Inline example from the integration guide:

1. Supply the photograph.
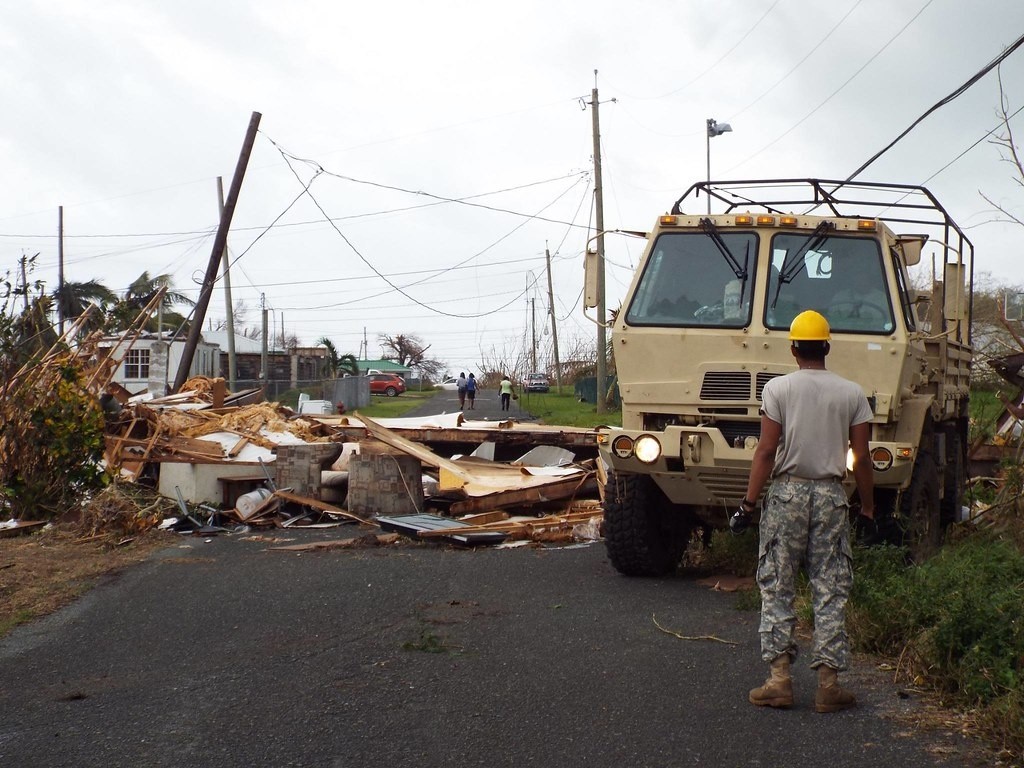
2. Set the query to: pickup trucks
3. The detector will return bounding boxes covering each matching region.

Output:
[523,373,550,393]
[342,370,383,378]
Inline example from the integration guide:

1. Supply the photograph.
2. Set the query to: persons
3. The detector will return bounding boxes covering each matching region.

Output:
[498,377,518,411]
[730,310,878,712]
[457,372,479,410]
[826,251,890,322]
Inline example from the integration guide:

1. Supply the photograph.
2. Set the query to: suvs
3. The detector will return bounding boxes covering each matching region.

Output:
[365,374,407,396]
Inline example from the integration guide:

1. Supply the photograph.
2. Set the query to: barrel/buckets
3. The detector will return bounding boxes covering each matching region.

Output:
[236,487,272,518]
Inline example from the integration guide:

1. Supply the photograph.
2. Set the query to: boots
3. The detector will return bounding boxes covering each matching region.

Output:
[749,655,793,707]
[815,665,856,712]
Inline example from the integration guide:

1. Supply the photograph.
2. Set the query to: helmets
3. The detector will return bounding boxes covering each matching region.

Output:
[787,310,831,340]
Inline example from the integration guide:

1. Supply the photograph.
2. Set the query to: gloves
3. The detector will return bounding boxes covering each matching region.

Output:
[853,514,875,542]
[729,505,754,536]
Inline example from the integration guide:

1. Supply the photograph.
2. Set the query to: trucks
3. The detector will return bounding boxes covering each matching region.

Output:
[584,176,975,578]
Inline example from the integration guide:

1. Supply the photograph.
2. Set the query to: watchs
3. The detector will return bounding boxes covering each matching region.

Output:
[742,496,756,507]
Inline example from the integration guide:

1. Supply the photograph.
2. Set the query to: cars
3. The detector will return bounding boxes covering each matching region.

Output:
[432,378,459,390]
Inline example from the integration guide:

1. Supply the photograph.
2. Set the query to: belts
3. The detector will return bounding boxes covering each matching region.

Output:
[778,473,834,483]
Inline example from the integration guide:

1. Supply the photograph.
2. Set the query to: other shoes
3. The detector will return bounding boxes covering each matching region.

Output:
[468,407,470,410]
[502,406,504,410]
[471,408,475,410]
[460,406,463,410]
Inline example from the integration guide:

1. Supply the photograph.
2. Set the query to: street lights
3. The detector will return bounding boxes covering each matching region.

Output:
[705,118,734,214]
